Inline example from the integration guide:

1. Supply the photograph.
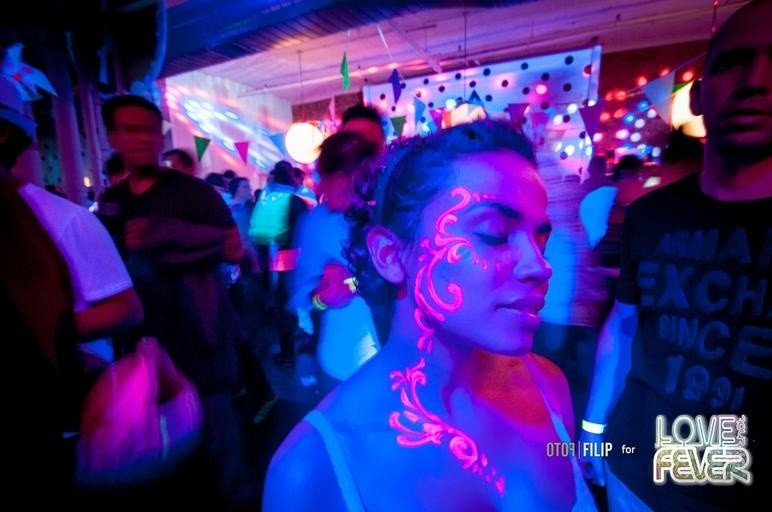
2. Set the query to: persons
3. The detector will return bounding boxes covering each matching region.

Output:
[1,0,772,511]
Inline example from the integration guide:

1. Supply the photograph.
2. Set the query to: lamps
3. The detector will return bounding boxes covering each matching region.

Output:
[284,51,323,163]
[442,12,488,128]
